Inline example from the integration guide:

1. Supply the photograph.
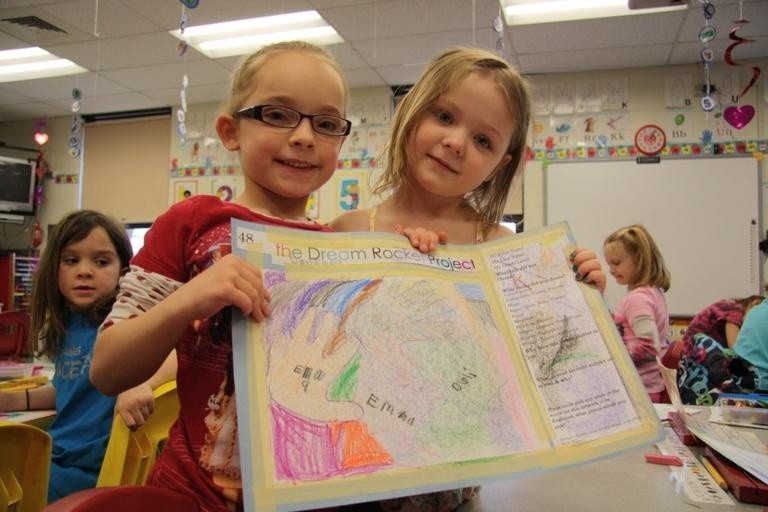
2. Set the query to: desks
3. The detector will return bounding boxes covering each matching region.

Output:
[471,403,767,512]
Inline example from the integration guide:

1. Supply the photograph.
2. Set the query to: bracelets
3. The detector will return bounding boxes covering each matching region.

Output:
[25,388,31,413]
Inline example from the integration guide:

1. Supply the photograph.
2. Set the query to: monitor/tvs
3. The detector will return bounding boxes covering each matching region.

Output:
[0,156,37,212]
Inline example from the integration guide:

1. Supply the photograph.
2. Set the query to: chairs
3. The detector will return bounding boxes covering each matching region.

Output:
[0,378,201,512]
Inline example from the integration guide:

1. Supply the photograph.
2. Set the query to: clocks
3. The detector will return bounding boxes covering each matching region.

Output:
[635,124,666,156]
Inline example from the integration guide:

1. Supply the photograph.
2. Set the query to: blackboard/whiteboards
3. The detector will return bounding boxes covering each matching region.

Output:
[545,156,760,316]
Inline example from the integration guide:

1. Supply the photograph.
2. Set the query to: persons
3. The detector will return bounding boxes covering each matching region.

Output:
[690,227,768,410]
[602,222,673,403]
[90,38,449,512]
[0,210,181,503]
[673,293,768,406]
[323,42,611,512]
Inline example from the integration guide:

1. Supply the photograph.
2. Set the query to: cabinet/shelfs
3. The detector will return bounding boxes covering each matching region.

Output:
[0,253,41,334]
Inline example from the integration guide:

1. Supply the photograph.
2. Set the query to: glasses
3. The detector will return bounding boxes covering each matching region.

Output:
[232,103,351,137]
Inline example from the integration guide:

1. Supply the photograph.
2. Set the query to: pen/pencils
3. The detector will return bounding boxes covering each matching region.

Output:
[697,454,728,494]
